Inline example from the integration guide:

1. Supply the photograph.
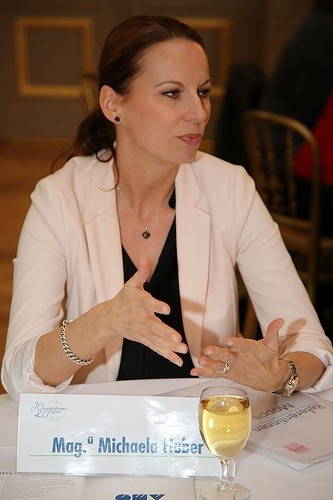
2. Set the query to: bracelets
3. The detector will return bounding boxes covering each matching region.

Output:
[59,319,95,365]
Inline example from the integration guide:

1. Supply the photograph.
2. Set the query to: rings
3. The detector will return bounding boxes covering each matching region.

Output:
[222,363,231,379]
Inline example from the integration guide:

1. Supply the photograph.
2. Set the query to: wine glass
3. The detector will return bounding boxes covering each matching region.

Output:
[198,386,251,500]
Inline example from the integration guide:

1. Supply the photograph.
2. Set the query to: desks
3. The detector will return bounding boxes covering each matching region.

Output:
[0,378,333,500]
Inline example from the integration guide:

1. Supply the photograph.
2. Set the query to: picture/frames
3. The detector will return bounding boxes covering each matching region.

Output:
[177,17,231,99]
[14,16,95,99]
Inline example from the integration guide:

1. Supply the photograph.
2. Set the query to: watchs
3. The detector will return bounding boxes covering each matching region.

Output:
[271,358,299,398]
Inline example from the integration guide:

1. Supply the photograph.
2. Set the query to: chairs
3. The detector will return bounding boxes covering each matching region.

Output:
[241,108,333,340]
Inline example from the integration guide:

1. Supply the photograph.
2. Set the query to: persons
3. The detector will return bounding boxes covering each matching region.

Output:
[1,13,332,405]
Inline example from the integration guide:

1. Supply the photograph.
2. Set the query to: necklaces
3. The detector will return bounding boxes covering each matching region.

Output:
[115,177,176,239]
